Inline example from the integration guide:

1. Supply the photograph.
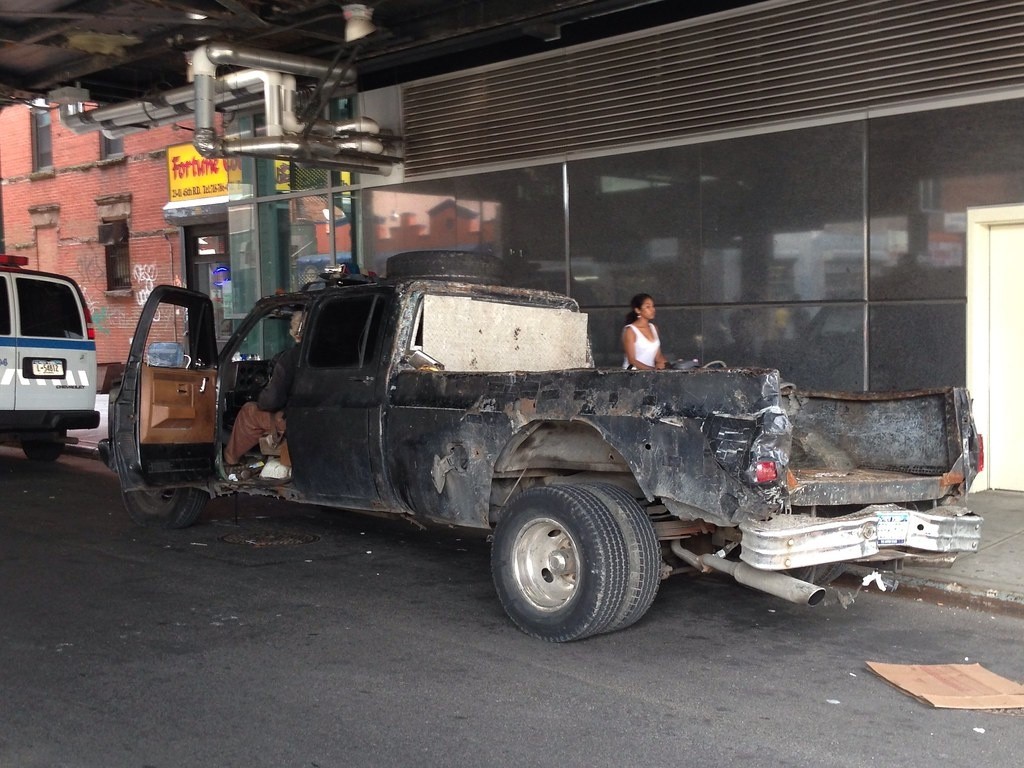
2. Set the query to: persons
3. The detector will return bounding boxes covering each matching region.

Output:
[220,310,307,468]
[621,294,669,370]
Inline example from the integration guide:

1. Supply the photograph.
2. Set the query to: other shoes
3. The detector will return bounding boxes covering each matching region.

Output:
[221,454,241,465]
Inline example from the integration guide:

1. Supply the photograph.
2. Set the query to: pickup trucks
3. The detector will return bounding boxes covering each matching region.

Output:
[97,250,987,645]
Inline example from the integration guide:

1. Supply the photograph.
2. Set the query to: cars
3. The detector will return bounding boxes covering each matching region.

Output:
[0,254,100,462]
